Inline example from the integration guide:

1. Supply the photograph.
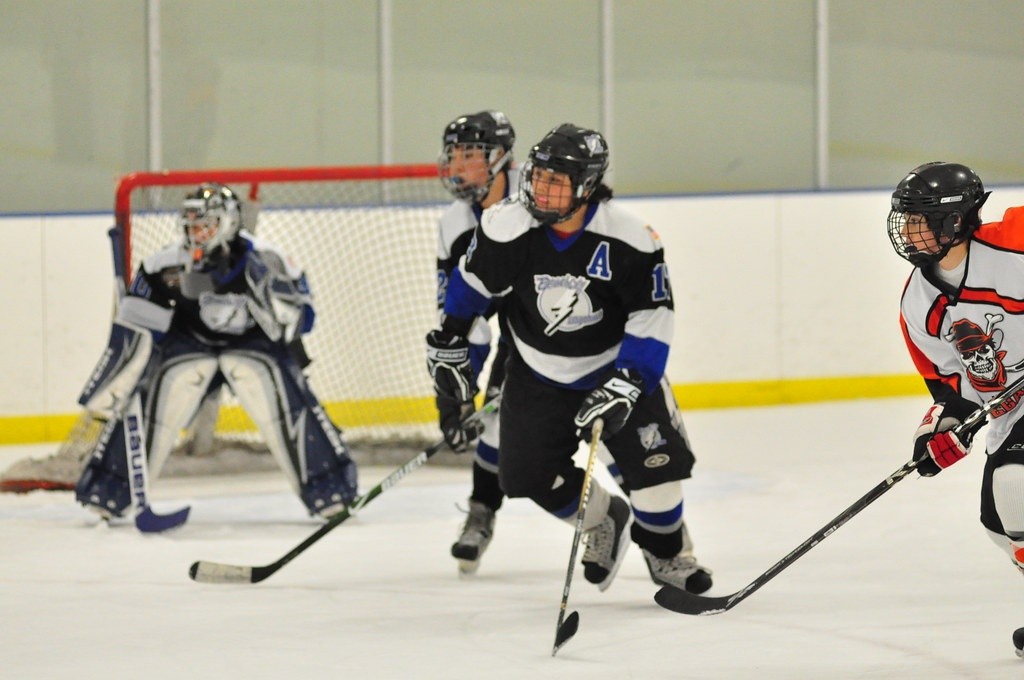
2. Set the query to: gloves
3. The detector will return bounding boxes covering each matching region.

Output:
[436,400,484,453]
[910,398,988,477]
[423,332,471,400]
[574,375,643,440]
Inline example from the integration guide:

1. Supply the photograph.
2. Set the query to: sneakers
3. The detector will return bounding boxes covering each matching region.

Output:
[452,495,495,574]
[643,550,714,595]
[582,493,635,592]
[1013,627,1024,656]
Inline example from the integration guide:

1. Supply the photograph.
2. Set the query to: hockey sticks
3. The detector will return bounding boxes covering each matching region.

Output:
[651,373,1024,619]
[550,416,606,659]
[186,394,502,589]
[107,223,192,535]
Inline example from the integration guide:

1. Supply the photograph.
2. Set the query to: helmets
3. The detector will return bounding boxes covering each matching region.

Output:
[438,110,514,202]
[177,183,240,260]
[887,162,991,269]
[518,123,609,223]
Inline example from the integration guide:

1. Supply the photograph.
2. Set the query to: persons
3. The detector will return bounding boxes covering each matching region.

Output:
[72,184,359,528]
[888,161,1024,661]
[426,123,713,594]
[437,111,631,580]
